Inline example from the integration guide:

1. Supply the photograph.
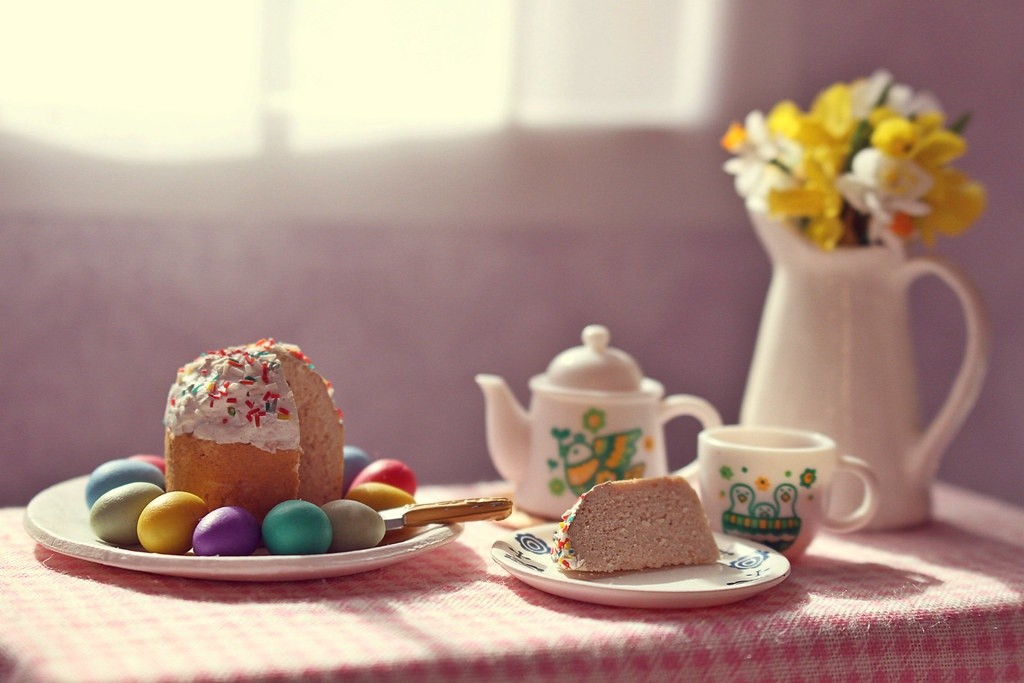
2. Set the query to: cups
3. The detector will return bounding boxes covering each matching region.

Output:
[696,425,881,562]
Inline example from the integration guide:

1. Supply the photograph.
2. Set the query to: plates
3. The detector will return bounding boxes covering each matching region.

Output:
[490,521,791,609]
[21,475,465,582]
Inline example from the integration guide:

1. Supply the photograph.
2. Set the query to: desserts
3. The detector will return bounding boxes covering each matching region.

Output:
[161,339,345,514]
[550,477,722,572]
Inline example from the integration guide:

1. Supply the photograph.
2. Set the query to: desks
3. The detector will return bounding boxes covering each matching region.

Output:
[1,482,1023,683]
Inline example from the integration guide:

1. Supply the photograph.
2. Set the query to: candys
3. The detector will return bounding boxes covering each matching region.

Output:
[84,446,418,557]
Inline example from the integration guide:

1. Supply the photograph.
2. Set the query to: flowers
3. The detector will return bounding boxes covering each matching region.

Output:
[718,71,990,249]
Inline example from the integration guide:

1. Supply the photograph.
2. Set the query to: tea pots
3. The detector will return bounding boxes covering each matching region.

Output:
[473,324,724,520]
